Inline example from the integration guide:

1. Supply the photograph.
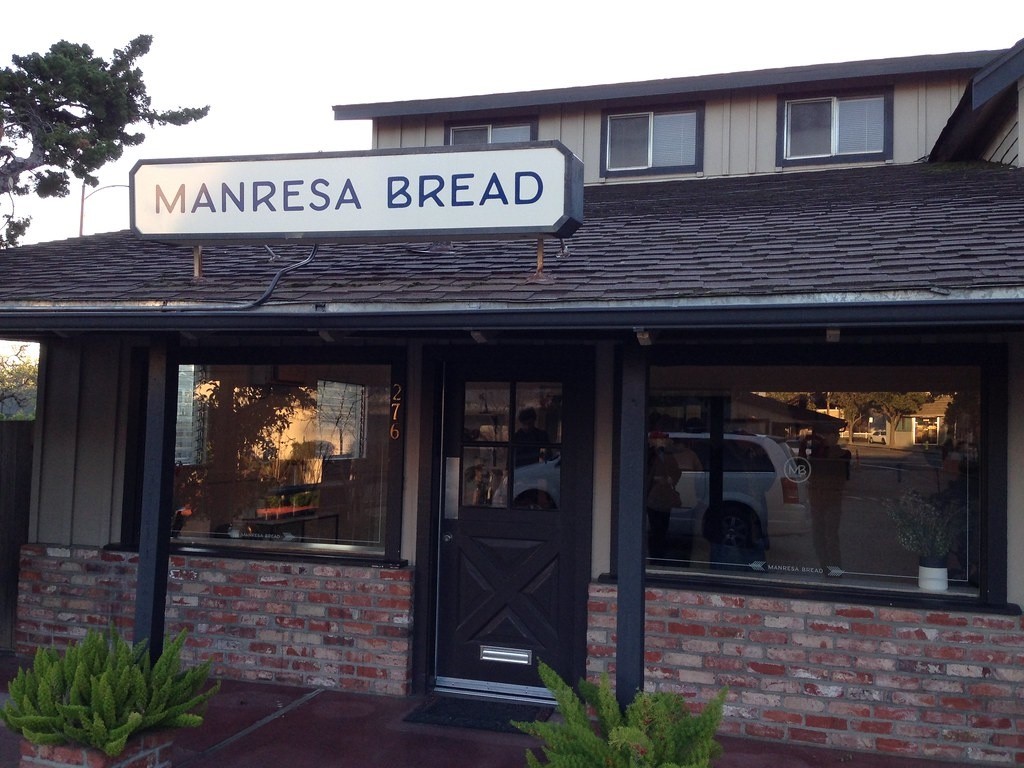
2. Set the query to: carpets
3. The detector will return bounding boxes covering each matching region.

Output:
[403,697,554,734]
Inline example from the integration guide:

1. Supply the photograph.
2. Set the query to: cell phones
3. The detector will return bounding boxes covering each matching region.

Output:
[806,435,818,440]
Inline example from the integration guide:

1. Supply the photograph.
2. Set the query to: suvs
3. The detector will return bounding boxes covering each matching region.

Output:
[489,431,811,564]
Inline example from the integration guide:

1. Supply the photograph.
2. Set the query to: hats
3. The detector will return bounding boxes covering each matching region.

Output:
[650,431,669,439]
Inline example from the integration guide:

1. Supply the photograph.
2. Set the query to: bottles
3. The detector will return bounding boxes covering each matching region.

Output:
[476,463,487,505]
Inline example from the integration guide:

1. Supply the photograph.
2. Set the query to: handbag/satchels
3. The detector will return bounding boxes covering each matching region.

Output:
[668,488,682,507]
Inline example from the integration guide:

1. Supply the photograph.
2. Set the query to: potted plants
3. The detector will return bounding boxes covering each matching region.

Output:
[878,491,972,592]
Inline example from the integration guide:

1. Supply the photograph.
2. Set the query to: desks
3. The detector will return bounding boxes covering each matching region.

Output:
[231,511,340,543]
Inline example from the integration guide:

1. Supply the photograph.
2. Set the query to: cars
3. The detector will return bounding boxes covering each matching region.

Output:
[868,430,888,445]
[787,439,812,456]
[173,461,239,519]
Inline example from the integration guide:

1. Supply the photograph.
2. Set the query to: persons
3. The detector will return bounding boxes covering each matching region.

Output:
[795,424,853,577]
[647,427,705,568]
[504,407,557,510]
